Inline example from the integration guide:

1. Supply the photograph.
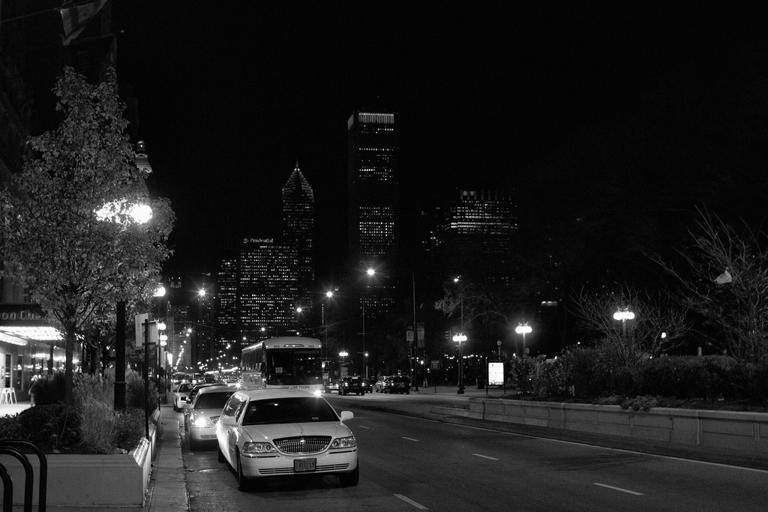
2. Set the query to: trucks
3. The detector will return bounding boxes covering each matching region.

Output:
[656,327,727,358]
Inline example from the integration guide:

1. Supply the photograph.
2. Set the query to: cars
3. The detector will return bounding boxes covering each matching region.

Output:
[336,375,409,396]
[215,388,359,490]
[171,371,240,452]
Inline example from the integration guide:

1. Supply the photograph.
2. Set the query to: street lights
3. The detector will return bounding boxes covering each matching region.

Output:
[92,195,152,416]
[514,321,533,356]
[613,303,635,339]
[153,282,174,404]
[453,272,467,387]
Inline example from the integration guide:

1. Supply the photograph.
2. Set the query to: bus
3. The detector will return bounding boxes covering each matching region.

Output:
[241,336,324,395]
